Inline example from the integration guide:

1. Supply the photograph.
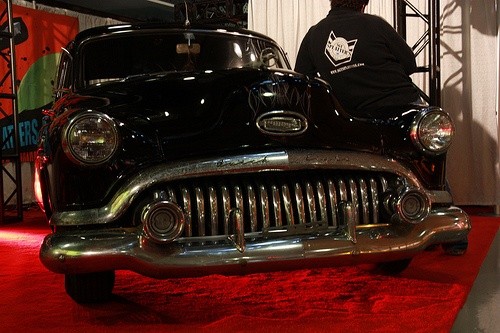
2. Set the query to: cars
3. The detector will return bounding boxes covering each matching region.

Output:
[32,19,473,304]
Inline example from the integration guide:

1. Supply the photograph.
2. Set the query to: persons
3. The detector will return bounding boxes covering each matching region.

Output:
[294,0,452,209]
